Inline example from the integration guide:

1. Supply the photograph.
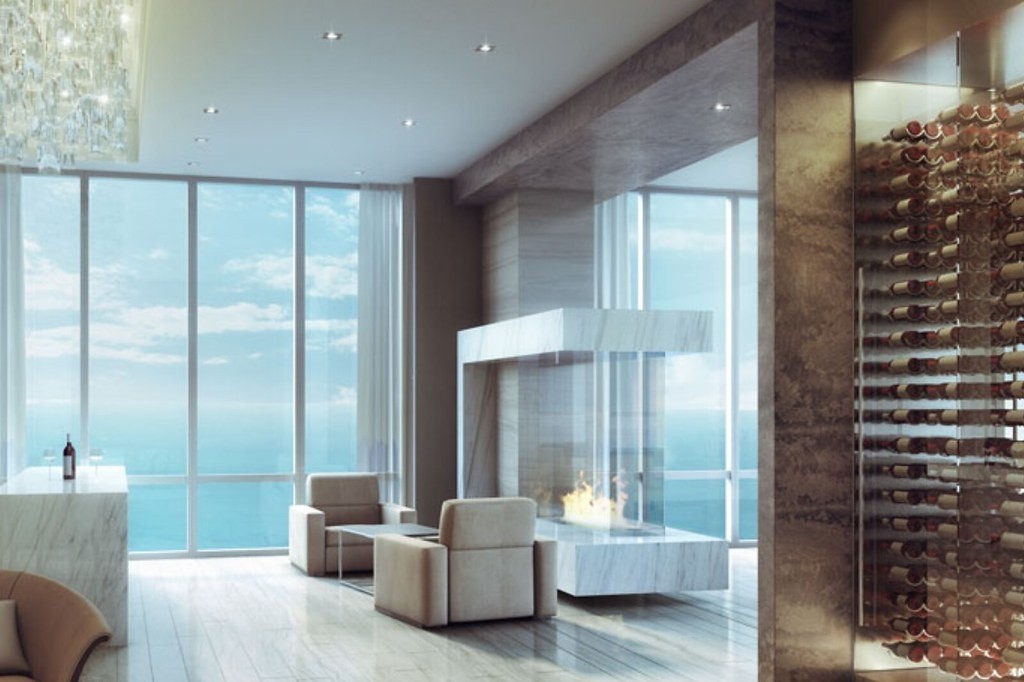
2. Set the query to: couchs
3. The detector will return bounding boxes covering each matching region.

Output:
[0,569,111,681]
[287,472,419,578]
[373,496,559,631]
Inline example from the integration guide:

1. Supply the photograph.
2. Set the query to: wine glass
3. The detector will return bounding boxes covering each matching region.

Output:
[88,447,105,478]
[44,447,58,479]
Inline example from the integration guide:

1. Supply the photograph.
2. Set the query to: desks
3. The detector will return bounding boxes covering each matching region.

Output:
[0,464,129,649]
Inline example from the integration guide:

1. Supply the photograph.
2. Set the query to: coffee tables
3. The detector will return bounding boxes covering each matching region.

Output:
[332,523,439,597]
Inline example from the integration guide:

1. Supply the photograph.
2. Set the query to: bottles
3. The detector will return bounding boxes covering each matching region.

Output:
[63,433,77,480]
[877,80,1024,681]
